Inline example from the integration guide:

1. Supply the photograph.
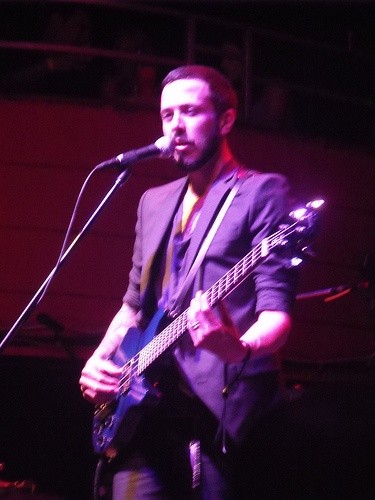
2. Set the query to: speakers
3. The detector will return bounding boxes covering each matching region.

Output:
[1,354,375,500]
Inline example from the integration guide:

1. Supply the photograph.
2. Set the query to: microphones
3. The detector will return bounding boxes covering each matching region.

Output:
[96,135,174,170]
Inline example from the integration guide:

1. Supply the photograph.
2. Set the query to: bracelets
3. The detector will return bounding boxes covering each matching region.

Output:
[214,339,252,454]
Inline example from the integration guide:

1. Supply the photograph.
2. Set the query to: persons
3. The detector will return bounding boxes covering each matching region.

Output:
[78,65,298,500]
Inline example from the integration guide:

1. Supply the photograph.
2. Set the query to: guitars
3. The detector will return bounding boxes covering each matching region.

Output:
[93,200,326,459]
